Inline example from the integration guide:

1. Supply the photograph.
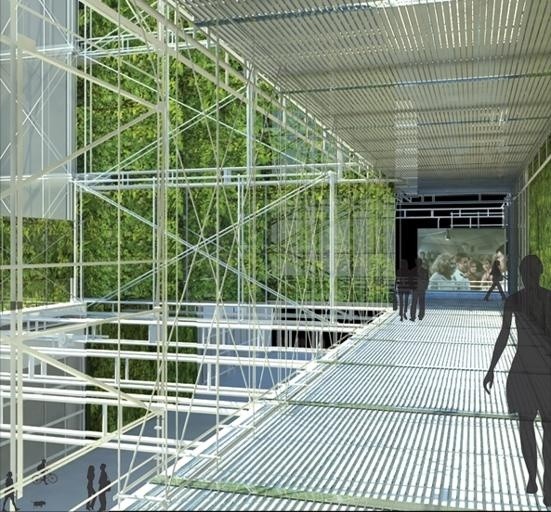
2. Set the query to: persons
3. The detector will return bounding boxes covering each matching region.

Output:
[420,241,509,291]
